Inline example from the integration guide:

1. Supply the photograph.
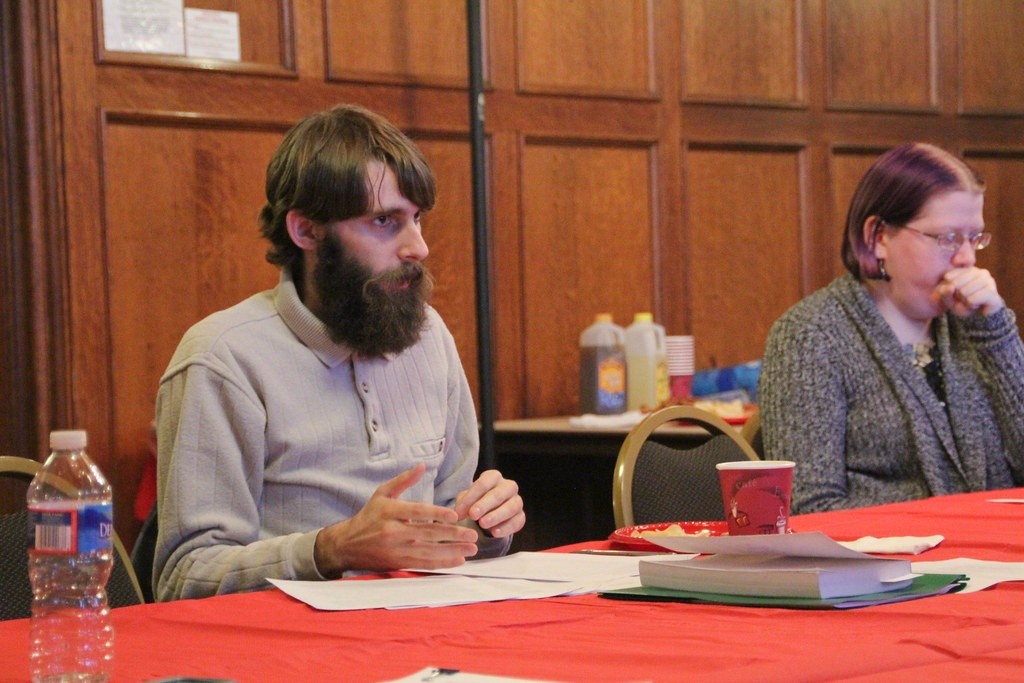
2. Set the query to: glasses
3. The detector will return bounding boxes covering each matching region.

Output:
[888,223,993,251]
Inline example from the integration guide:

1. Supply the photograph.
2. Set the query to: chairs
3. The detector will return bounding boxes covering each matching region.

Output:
[0,456,147,621]
[611,404,764,525]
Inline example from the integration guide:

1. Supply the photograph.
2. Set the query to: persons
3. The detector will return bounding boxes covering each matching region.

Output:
[151,103,525,603]
[758,141,1024,516]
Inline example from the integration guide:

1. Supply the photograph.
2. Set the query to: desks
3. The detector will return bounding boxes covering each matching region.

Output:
[494,414,756,556]
[0,485,1024,683]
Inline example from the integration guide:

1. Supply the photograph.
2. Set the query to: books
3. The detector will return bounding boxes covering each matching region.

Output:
[640,531,913,599]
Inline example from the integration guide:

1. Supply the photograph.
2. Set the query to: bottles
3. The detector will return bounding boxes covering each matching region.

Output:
[27,428,116,683]
[579,312,671,414]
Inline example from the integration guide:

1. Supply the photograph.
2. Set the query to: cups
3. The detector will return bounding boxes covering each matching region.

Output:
[664,334,695,405]
[715,460,797,534]
